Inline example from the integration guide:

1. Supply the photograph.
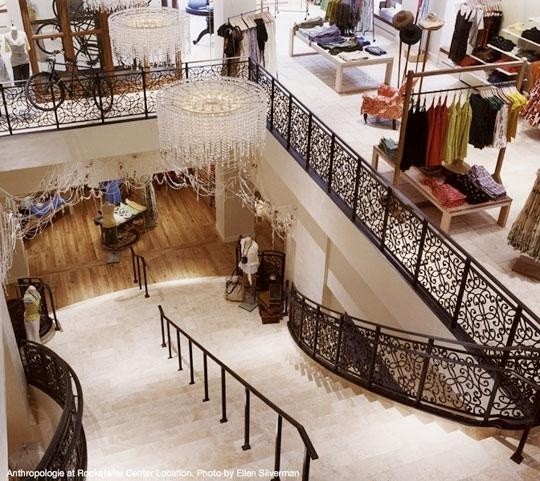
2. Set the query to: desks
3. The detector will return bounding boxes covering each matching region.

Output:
[369,138,514,232]
[185,0,214,44]
[288,18,394,93]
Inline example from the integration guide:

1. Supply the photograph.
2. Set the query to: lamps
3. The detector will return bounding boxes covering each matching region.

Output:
[107,0,192,70]
[81,0,150,16]
[157,0,267,202]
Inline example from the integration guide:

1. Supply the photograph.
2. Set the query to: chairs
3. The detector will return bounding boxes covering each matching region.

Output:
[360,74,419,129]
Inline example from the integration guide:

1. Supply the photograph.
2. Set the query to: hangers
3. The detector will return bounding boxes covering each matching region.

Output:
[472,80,527,106]
[459,0,503,15]
[226,6,275,32]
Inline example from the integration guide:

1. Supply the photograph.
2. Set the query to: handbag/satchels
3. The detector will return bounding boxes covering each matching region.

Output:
[473,47,501,63]
[491,35,516,51]
[460,57,476,66]
[521,27,540,44]
[487,72,512,88]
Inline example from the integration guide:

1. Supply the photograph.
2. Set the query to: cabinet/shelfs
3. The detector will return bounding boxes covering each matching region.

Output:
[458,16,540,94]
[94,197,147,251]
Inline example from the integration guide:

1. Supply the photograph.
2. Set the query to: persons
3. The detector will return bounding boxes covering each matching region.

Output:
[3,24,32,87]
[191,7,214,44]
[100,200,120,263]
[27,189,66,217]
[20,285,42,351]
[237,235,262,312]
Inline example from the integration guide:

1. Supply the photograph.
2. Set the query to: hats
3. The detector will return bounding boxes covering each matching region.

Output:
[399,23,422,44]
[419,12,444,31]
[393,11,414,29]
